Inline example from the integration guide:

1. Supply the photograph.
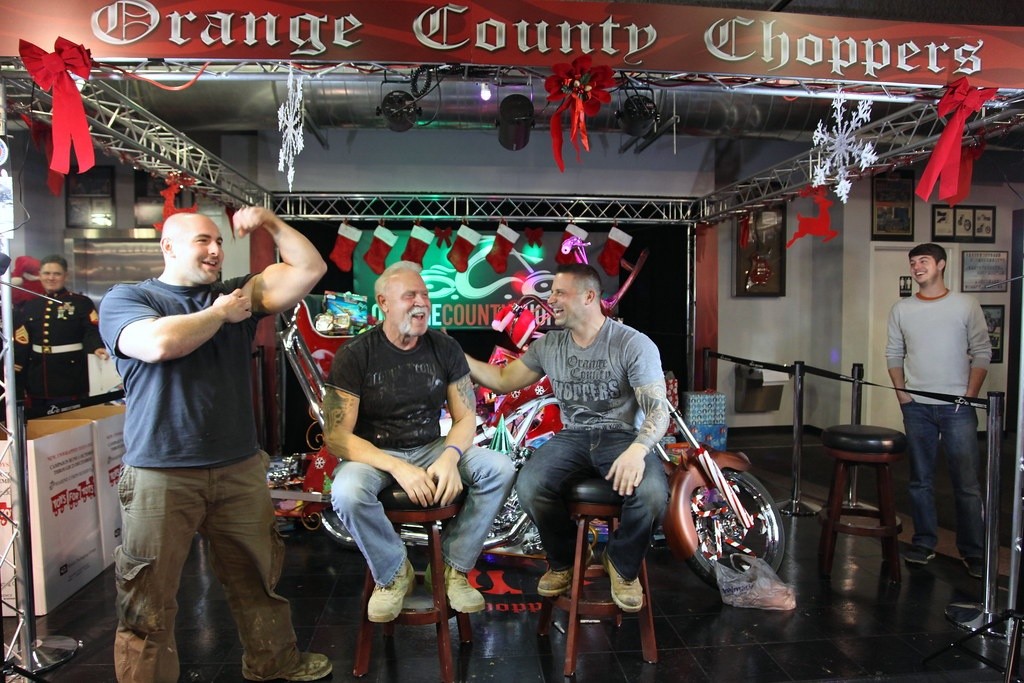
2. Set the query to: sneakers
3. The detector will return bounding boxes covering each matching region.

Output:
[537,544,596,597]
[424,560,486,613]
[243,652,332,681]
[368,557,415,622]
[602,547,643,613]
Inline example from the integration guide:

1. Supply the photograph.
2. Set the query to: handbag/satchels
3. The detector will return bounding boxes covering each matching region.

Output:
[711,553,796,611]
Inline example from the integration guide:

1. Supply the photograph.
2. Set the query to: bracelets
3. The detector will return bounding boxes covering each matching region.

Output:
[444,445,463,465]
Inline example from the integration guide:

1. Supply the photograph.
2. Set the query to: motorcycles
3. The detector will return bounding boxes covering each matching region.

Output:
[278,324,787,589]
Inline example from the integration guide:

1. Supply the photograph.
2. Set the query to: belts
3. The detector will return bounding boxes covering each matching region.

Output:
[32,343,83,353]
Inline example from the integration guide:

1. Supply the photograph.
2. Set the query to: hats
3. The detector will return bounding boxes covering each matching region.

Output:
[492,305,535,348]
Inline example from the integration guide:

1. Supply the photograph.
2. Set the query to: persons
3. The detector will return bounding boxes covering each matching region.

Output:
[884,243,992,577]
[13,255,110,420]
[323,261,517,623]
[440,262,673,613]
[98,206,334,683]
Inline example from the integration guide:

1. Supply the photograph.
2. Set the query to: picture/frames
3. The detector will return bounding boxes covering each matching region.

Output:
[931,203,996,244]
[871,169,915,242]
[961,250,1008,293]
[967,304,1005,364]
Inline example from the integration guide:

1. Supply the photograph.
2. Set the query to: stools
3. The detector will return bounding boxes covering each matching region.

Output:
[816,423,907,583]
[351,478,472,683]
[535,469,659,677]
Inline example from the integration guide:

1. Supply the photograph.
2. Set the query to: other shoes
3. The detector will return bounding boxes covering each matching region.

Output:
[902,546,936,564]
[962,557,983,581]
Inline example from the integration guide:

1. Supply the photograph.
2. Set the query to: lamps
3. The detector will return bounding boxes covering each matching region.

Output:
[620,87,661,137]
[495,81,533,152]
[375,79,422,132]
